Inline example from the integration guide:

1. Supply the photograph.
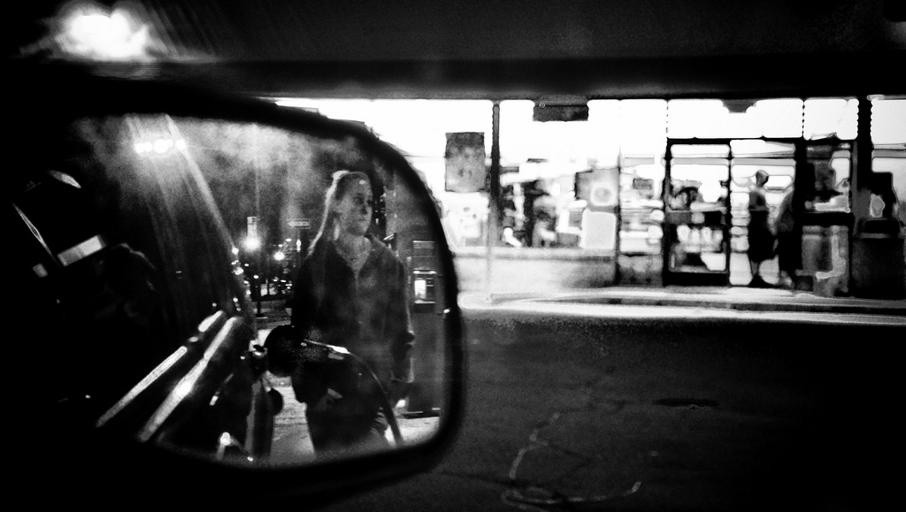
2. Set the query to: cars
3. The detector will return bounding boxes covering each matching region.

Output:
[15,110,279,462]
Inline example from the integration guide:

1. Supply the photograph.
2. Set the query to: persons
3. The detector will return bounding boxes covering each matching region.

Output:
[290,170,414,454]
[747,170,774,288]
[774,190,814,288]
